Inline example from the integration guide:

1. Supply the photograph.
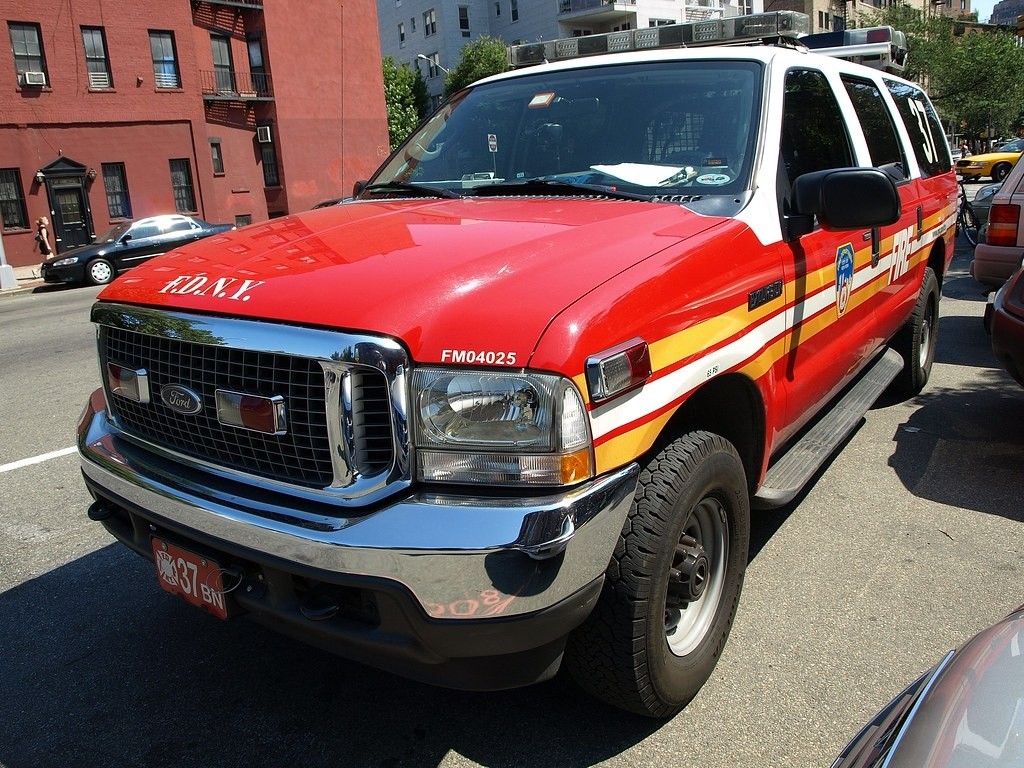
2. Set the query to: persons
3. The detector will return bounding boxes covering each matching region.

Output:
[699,96,792,212]
[32,217,54,279]
[961,140,986,182]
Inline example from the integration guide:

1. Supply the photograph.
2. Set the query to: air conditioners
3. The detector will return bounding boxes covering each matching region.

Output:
[23,72,46,86]
[257,126,273,143]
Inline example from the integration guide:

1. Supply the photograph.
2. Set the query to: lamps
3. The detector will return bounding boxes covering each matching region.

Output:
[88,167,98,180]
[36,170,45,183]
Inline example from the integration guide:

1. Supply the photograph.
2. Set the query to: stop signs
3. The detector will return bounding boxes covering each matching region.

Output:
[489,135,498,152]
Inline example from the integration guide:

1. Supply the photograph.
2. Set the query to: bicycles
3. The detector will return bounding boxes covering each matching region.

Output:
[956,174,983,247]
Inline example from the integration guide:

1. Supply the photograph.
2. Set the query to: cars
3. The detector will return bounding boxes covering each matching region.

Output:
[970,151,1024,386]
[957,139,1024,182]
[830,603,1024,768]
[41,215,238,285]
[952,147,972,164]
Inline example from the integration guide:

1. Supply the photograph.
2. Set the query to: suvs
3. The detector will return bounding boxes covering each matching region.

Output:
[76,9,960,717]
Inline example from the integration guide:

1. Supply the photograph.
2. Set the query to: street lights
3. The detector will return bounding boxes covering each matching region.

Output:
[418,54,449,79]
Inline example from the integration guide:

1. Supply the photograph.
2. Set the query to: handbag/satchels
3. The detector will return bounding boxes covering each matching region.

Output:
[34,228,44,242]
